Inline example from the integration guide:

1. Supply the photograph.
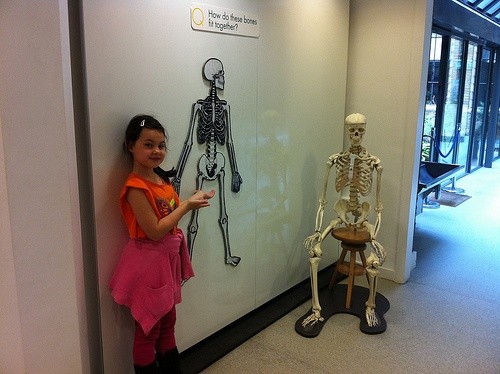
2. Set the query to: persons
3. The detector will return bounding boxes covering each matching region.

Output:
[105,114,216,374]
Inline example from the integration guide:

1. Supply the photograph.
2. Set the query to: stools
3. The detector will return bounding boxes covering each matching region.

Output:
[328,227,371,309]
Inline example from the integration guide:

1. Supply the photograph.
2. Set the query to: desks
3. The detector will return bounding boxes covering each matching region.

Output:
[418,161,465,196]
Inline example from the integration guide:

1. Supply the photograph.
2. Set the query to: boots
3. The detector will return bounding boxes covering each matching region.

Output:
[134,347,184,374]
